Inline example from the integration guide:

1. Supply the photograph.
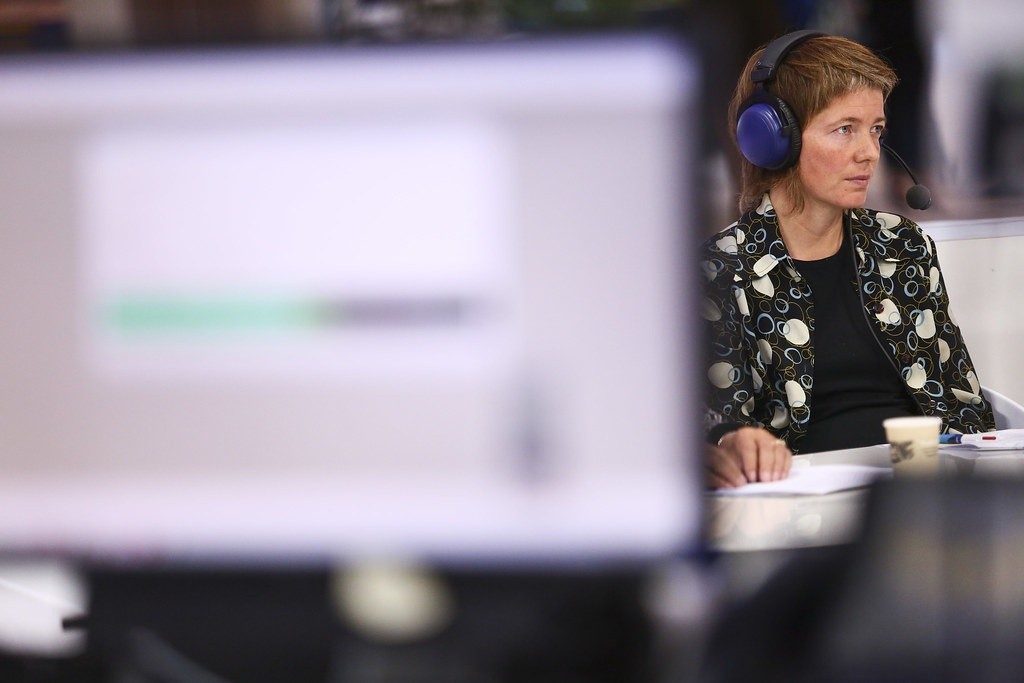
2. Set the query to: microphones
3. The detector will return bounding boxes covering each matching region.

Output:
[879,139,932,211]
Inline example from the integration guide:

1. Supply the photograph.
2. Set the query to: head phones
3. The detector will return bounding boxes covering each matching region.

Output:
[737,29,834,170]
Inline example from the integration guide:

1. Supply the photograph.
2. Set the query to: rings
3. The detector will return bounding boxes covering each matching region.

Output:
[774,440,785,446]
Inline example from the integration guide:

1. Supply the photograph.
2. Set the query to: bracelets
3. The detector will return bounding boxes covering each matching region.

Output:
[718,438,722,446]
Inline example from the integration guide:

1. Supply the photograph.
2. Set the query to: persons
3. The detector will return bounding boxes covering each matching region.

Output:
[701,400,792,487]
[701,33,997,455]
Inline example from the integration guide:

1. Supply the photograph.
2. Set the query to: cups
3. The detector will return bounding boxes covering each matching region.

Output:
[880,413,944,483]
